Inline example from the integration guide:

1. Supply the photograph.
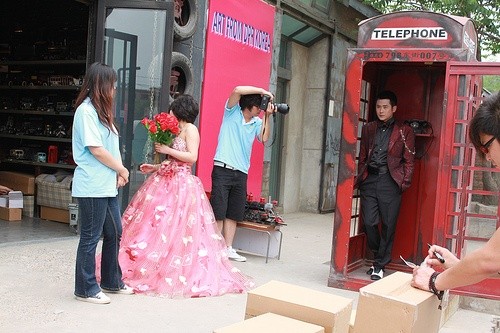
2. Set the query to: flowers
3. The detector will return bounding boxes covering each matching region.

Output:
[141,113,182,167]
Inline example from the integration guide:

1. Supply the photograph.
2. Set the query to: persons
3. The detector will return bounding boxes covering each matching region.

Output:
[209,85,278,262]
[353,91,416,280]
[70,63,135,304]
[95,93,259,300]
[410,92,500,293]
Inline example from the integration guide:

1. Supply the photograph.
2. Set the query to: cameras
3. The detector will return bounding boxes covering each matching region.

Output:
[260,94,289,114]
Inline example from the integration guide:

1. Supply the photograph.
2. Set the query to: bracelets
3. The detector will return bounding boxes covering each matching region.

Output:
[429,272,444,310]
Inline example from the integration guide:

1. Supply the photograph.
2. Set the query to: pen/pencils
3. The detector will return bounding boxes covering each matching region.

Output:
[427,243,445,263]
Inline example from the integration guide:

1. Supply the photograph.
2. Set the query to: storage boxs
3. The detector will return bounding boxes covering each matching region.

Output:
[0,170,76,223]
[244,280,353,333]
[213,312,325,333]
[351,271,443,333]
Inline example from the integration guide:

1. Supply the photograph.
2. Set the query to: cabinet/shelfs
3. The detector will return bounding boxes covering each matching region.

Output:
[0,59,85,217]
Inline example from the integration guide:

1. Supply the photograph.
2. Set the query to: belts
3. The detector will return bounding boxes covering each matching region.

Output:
[214,162,238,170]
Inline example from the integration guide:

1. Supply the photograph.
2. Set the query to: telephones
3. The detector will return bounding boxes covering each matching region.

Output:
[405,119,431,134]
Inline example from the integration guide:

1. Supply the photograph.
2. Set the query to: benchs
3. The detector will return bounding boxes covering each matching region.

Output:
[240,221,283,263]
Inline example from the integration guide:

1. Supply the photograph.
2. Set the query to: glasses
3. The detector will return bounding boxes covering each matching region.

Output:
[479,134,498,155]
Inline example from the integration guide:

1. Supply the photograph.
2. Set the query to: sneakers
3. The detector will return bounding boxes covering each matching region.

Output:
[101,284,134,295]
[367,266,374,275]
[75,291,111,304]
[371,268,384,280]
[226,246,246,262]
